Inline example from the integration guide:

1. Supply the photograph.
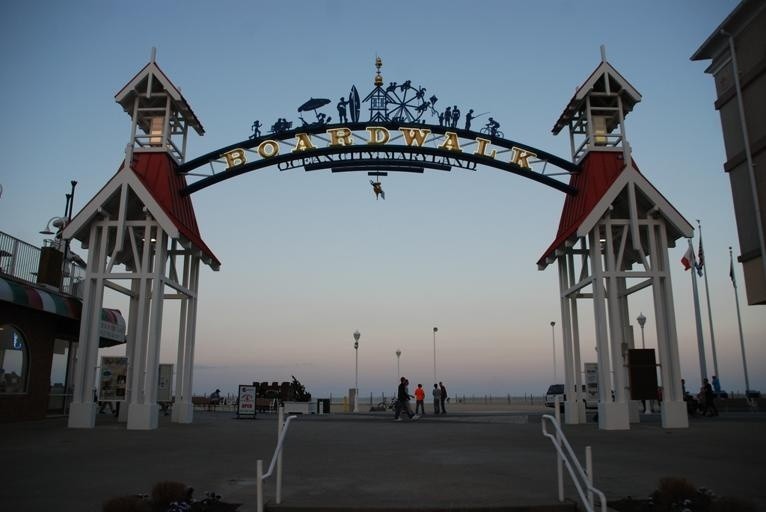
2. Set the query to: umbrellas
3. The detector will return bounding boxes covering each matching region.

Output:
[0,249,12,258]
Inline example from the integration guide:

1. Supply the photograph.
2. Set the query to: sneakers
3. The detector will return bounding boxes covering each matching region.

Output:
[409,414,423,422]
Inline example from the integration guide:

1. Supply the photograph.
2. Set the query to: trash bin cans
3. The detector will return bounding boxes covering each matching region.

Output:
[317,398,330,415]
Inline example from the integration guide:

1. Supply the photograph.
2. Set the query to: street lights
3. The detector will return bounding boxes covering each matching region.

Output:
[38,214,66,291]
[432,327,439,383]
[395,350,402,398]
[352,329,360,388]
[550,320,557,383]
[636,311,647,349]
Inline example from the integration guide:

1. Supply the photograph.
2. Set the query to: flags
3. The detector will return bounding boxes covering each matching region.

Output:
[683,241,703,278]
[729,256,737,290]
[697,231,705,277]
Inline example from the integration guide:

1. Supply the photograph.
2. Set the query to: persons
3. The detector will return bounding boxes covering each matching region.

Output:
[393,375,450,423]
[711,375,722,392]
[251,98,501,140]
[681,378,702,418]
[208,388,220,412]
[700,377,720,417]
[641,386,663,413]
[98,400,117,414]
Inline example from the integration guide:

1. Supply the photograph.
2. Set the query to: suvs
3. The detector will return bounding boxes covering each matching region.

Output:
[545,383,586,410]
